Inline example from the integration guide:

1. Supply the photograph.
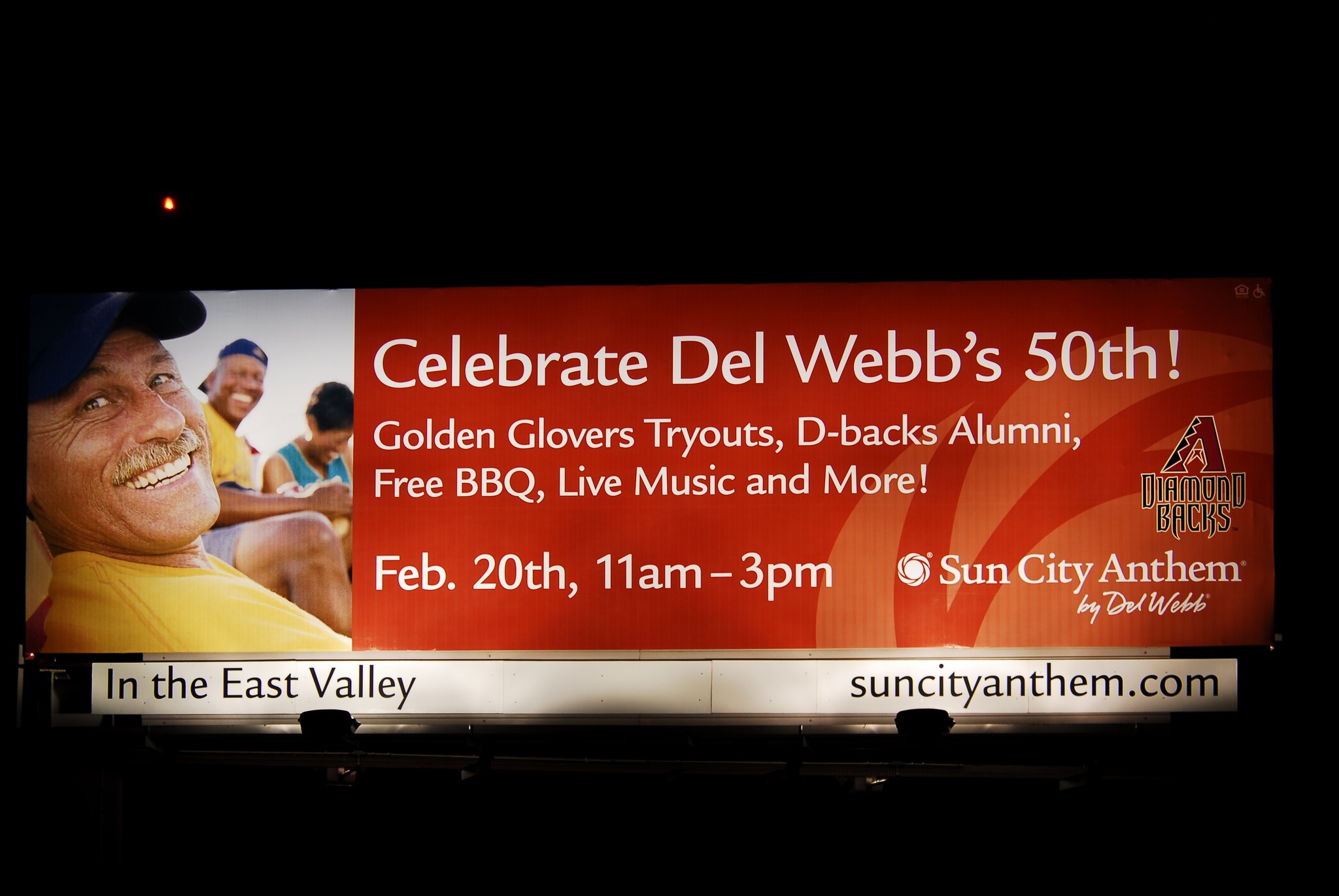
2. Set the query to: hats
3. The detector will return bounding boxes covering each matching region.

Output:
[200,335,266,394]
[28,290,207,406]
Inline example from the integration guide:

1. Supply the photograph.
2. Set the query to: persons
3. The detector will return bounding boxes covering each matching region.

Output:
[201,338,354,638]
[24,291,353,652]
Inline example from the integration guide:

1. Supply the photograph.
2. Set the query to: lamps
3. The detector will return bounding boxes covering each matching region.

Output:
[895,709,955,735]
[298,710,360,734]
